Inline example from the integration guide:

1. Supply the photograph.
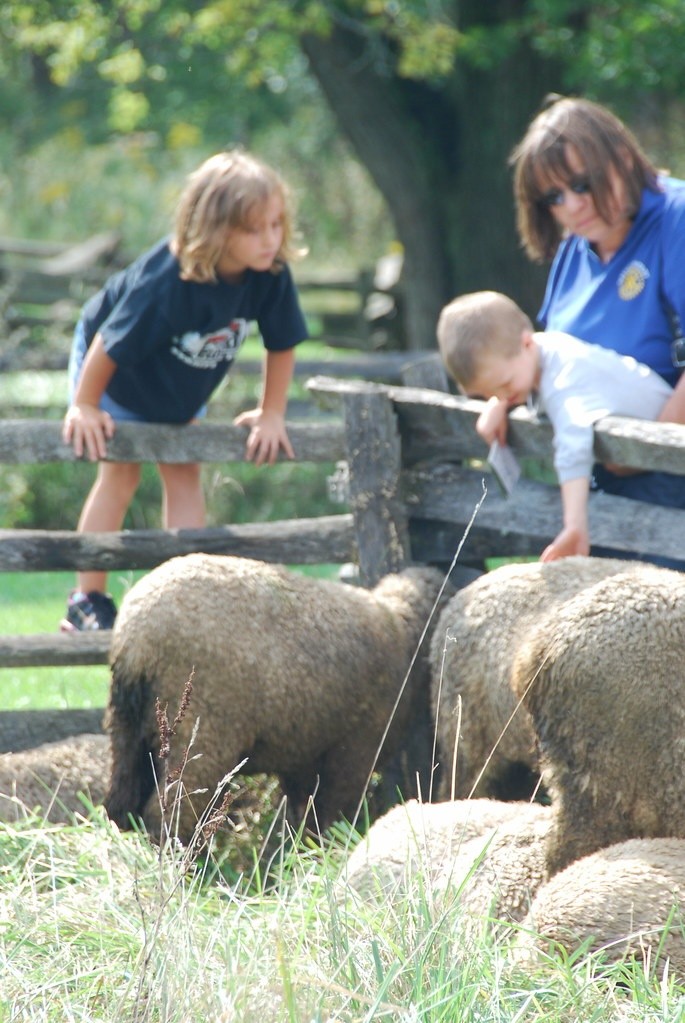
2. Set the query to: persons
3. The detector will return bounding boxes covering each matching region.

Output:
[61,152,308,632]
[514,98,685,571]
[437,291,676,563]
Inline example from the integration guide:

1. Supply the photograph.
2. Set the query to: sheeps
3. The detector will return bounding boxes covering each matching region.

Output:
[95,553,684,998]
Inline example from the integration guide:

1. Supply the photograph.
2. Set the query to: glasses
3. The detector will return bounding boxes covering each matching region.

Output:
[535,169,607,214]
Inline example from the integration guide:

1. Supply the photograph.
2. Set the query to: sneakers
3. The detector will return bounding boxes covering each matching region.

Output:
[58,588,117,632]
